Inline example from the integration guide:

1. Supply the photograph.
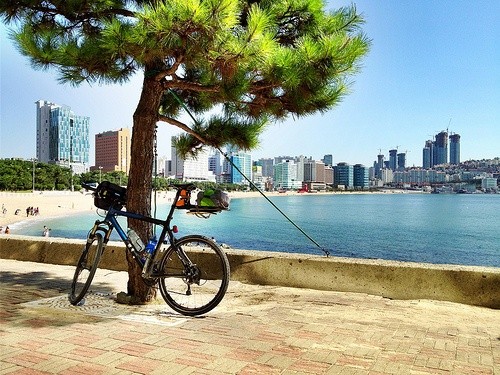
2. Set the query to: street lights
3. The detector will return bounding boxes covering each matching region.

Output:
[99,166,104,183]
[31,157,39,190]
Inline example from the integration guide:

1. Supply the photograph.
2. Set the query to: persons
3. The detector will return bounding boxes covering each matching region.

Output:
[43,226,50,236]
[14,209,22,215]
[5,226,11,235]
[2,203,7,210]
[3,208,8,214]
[26,206,39,217]
[0,226,4,234]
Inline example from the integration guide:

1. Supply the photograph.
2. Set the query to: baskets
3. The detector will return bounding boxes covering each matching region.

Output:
[93,184,127,211]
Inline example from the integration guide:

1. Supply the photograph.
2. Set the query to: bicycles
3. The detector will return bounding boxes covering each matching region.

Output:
[69,180,231,316]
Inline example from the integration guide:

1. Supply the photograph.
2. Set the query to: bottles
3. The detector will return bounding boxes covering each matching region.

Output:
[126,228,145,252]
[141,235,157,262]
